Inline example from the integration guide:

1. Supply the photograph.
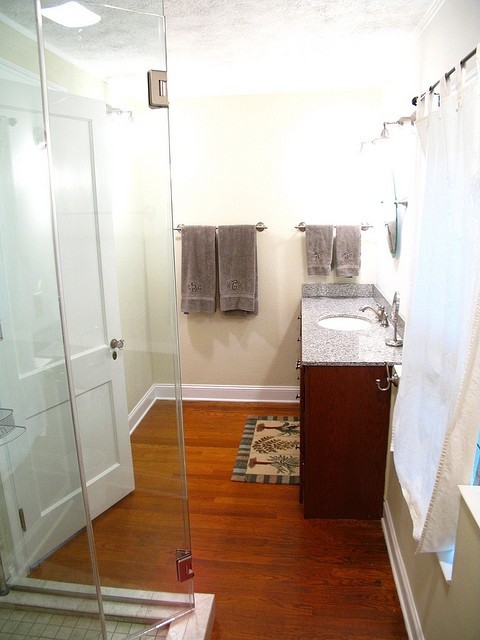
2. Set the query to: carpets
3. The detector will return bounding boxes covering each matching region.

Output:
[231,416,300,484]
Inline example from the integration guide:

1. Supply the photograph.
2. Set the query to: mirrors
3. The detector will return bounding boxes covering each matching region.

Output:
[375,116,420,322]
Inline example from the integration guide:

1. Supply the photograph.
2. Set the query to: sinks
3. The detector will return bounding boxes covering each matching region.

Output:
[315,313,369,333]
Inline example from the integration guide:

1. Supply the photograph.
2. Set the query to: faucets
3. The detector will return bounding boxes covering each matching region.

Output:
[359,303,385,321]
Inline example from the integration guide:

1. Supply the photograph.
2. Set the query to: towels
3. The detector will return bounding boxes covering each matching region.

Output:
[181,226,217,313]
[387,218,401,260]
[335,225,362,279]
[305,225,334,275]
[218,226,258,312]
